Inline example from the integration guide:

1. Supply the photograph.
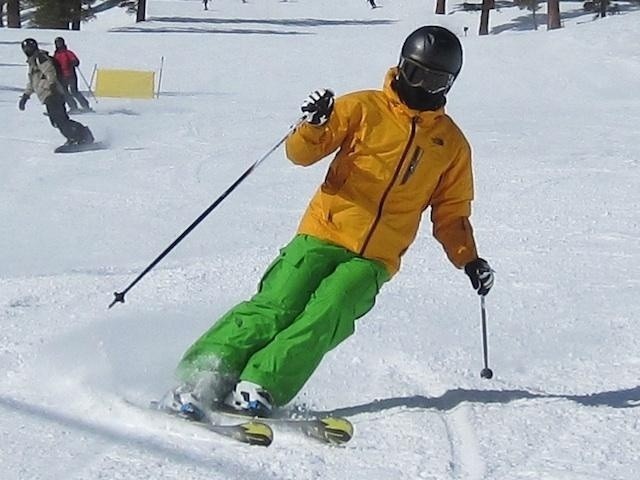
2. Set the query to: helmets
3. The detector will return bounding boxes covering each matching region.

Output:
[21,38,39,56]
[398,25,465,93]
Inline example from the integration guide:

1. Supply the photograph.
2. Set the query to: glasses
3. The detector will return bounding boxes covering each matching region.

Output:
[401,55,453,96]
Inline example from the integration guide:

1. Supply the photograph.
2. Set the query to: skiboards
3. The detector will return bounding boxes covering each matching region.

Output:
[123,379,353,447]
[43,108,97,115]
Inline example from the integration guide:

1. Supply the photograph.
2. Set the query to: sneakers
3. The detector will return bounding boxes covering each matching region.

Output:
[216,378,276,418]
[164,373,221,421]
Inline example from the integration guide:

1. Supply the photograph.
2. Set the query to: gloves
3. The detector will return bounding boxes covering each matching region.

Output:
[300,88,335,130]
[465,259,496,299]
[17,92,29,110]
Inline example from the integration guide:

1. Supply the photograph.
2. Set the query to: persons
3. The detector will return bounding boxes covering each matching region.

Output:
[52,36,90,114]
[165,22,497,422]
[17,37,95,147]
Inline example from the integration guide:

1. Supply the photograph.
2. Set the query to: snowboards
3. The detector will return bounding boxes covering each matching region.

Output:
[54,142,110,152]
